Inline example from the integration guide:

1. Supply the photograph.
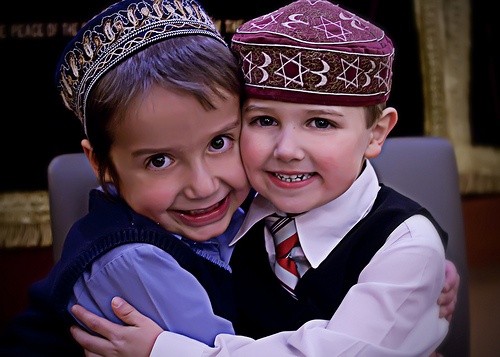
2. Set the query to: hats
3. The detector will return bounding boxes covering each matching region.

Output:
[57,0,225,140]
[230,0,395,107]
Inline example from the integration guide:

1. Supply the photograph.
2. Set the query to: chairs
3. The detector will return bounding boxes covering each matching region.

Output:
[48,139,475,357]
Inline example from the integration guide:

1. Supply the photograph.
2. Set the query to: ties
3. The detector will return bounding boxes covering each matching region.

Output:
[260,214,306,299]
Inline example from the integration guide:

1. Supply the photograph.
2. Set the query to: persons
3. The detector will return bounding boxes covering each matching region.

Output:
[0,1,460,357]
[70,0,450,357]
